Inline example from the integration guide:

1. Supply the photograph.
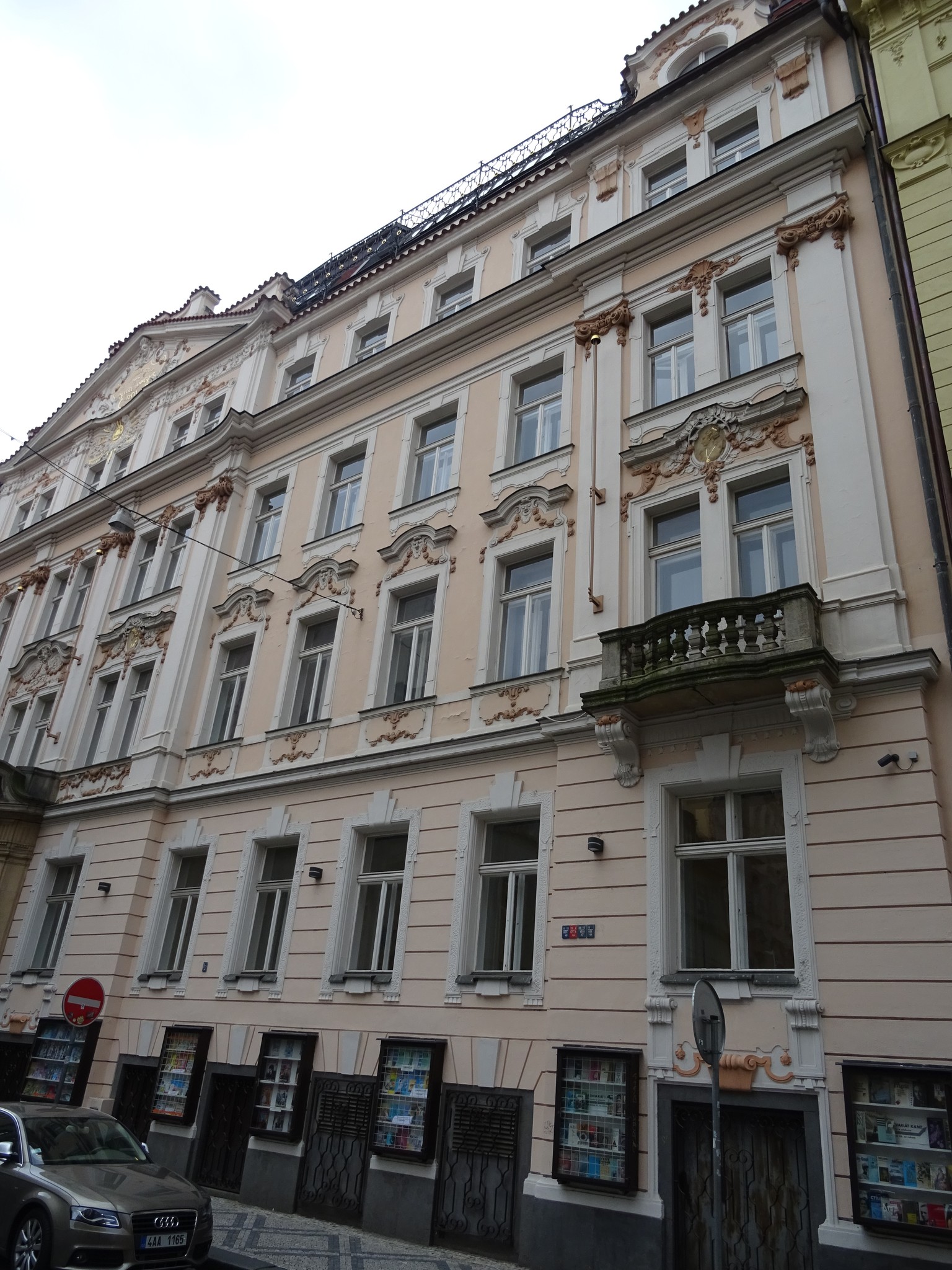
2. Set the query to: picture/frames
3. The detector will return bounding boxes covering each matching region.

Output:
[151,1024,214,1127]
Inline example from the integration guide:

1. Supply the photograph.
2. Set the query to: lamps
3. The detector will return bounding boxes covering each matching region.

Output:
[309,867,323,878]
[588,837,603,854]
[108,507,135,533]
[98,882,111,892]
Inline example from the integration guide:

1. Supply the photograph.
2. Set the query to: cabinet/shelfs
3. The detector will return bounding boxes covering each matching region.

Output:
[550,1045,641,1196]
[15,1017,103,1107]
[372,1035,448,1164]
[835,1060,952,1241]
[247,1030,319,1143]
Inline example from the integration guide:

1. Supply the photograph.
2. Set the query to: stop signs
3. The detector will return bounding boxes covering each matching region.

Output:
[61,976,106,1028]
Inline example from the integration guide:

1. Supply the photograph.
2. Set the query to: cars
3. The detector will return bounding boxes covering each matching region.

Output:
[0,1100,213,1270]
